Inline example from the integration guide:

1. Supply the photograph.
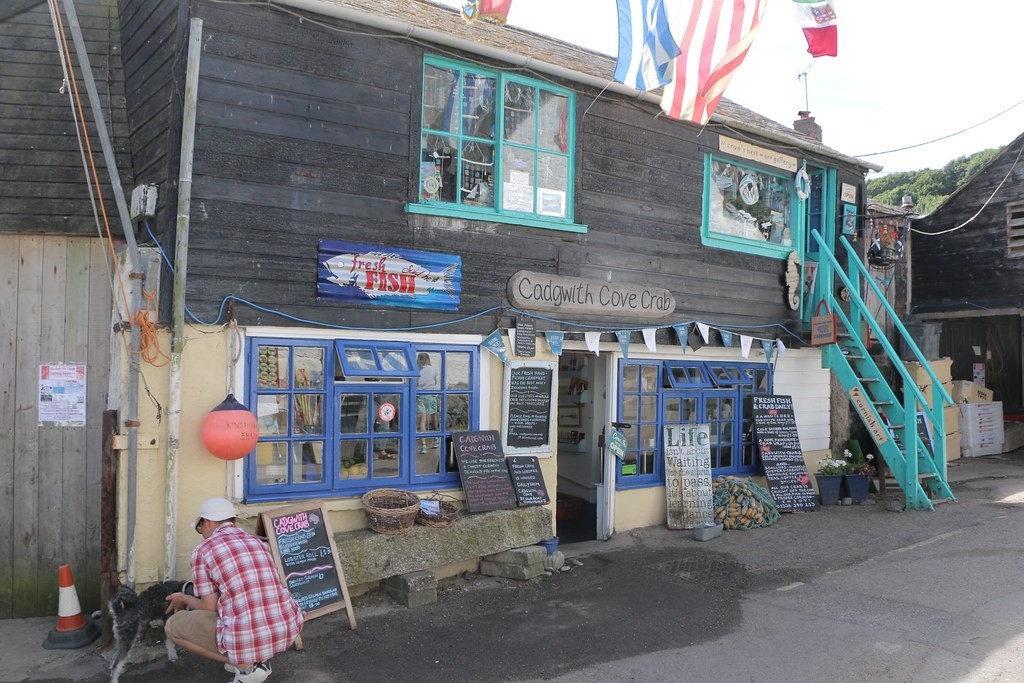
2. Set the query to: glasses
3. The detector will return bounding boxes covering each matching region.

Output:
[195,519,207,535]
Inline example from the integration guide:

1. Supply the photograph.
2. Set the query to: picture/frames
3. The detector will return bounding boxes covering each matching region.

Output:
[558,404,581,428]
[537,188,565,217]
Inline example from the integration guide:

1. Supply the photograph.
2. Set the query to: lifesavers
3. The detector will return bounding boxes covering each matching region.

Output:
[795,169,810,200]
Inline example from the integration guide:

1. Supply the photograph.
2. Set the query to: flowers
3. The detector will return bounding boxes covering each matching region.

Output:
[817,448,877,478]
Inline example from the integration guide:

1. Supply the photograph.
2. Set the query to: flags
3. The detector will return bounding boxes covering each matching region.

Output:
[443,71,493,150]
[659,0,769,125]
[614,0,682,92]
[793,0,837,58]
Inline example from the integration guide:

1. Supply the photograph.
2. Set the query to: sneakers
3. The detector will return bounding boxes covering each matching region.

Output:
[231,662,272,683]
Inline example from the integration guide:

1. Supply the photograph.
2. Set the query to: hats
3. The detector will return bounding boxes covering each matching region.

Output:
[198,497,241,521]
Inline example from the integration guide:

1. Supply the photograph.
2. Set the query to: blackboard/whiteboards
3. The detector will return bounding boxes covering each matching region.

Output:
[255,499,351,621]
[516,317,536,357]
[500,360,556,458]
[752,394,821,512]
[505,455,551,506]
[452,430,518,513]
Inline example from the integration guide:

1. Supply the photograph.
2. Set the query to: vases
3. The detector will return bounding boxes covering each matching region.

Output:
[843,475,871,504]
[814,472,842,506]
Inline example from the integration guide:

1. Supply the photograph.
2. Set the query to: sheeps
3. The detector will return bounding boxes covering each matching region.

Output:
[92,579,201,683]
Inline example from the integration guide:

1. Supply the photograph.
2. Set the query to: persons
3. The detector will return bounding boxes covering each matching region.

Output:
[417,353,439,454]
[164,497,304,683]
[352,395,397,464]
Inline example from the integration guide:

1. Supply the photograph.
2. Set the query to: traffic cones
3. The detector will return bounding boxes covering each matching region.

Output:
[43,563,101,651]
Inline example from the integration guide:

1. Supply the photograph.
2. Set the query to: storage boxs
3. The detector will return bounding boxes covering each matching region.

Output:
[898,321,1024,465]
